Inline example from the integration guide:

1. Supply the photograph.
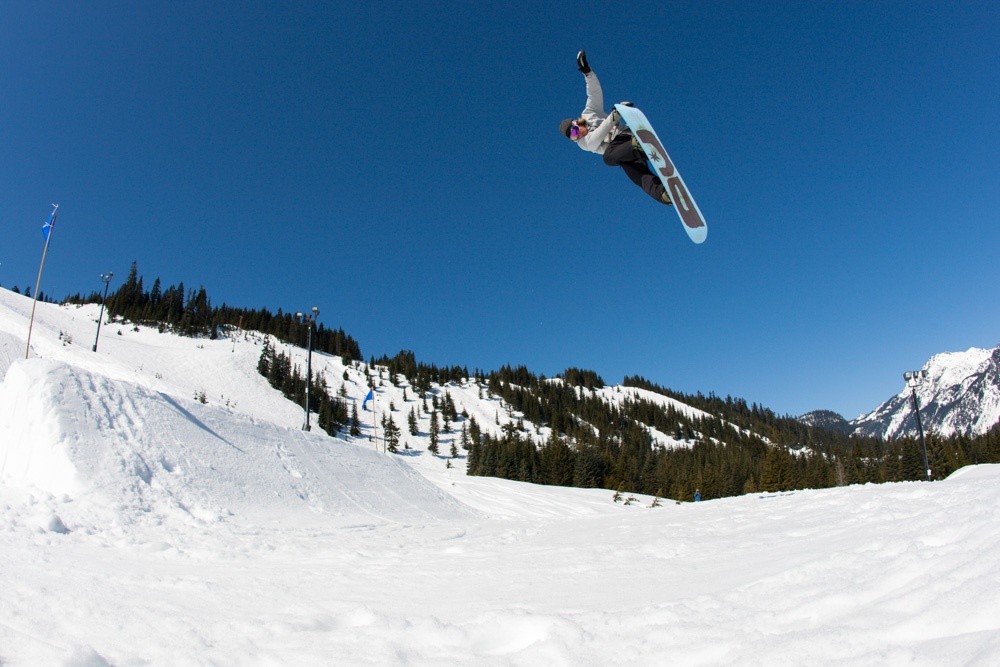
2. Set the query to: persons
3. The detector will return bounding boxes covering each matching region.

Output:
[560,50,674,205]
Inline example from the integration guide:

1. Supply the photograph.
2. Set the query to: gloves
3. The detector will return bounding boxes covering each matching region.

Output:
[613,102,634,122]
[576,50,591,74]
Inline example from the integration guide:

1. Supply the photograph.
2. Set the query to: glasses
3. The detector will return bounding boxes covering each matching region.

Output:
[569,125,579,141]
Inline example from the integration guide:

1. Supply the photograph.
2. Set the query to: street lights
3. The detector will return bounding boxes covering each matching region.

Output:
[95,271,115,352]
[903,370,934,480]
[296,306,320,430]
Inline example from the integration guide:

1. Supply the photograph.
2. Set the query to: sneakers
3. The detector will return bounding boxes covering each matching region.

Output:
[661,191,673,206]
[630,133,643,152]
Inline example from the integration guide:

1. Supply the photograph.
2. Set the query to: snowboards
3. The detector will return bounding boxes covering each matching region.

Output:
[613,102,710,245]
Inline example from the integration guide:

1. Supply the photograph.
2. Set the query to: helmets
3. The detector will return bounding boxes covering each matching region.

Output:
[559,118,573,140]
